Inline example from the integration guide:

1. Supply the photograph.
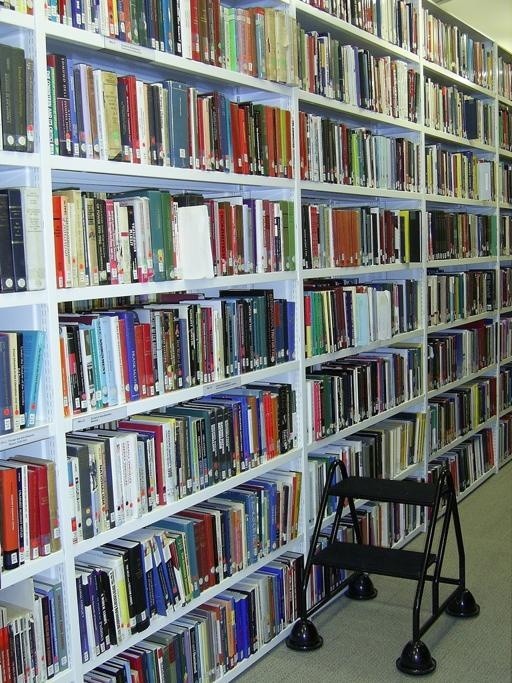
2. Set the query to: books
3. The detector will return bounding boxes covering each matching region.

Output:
[295,20,492,146]
[426,425,496,518]
[417,375,498,454]
[304,411,428,528]
[0,47,40,153]
[498,367,512,414]
[0,182,45,294]
[421,141,495,203]
[302,199,423,274]
[427,209,500,259]
[302,277,431,357]
[498,54,512,100]
[491,414,512,466]
[297,109,421,192]
[1,451,62,577]
[54,182,298,289]
[500,318,512,364]
[310,476,428,609]
[1,580,71,683]
[43,50,293,179]
[2,0,294,93]
[305,339,426,441]
[75,469,301,661]
[496,107,512,151]
[498,161,512,207]
[82,547,310,681]
[499,265,512,314]
[419,323,496,390]
[0,327,47,437]
[55,289,299,416]
[429,269,499,328]
[501,213,512,259]
[298,0,512,102]
[64,381,293,548]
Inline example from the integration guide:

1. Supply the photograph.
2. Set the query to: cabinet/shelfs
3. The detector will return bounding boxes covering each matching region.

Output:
[0,1,512,683]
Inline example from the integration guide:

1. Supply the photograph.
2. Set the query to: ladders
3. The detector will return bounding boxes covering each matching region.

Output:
[286,460,480,674]
